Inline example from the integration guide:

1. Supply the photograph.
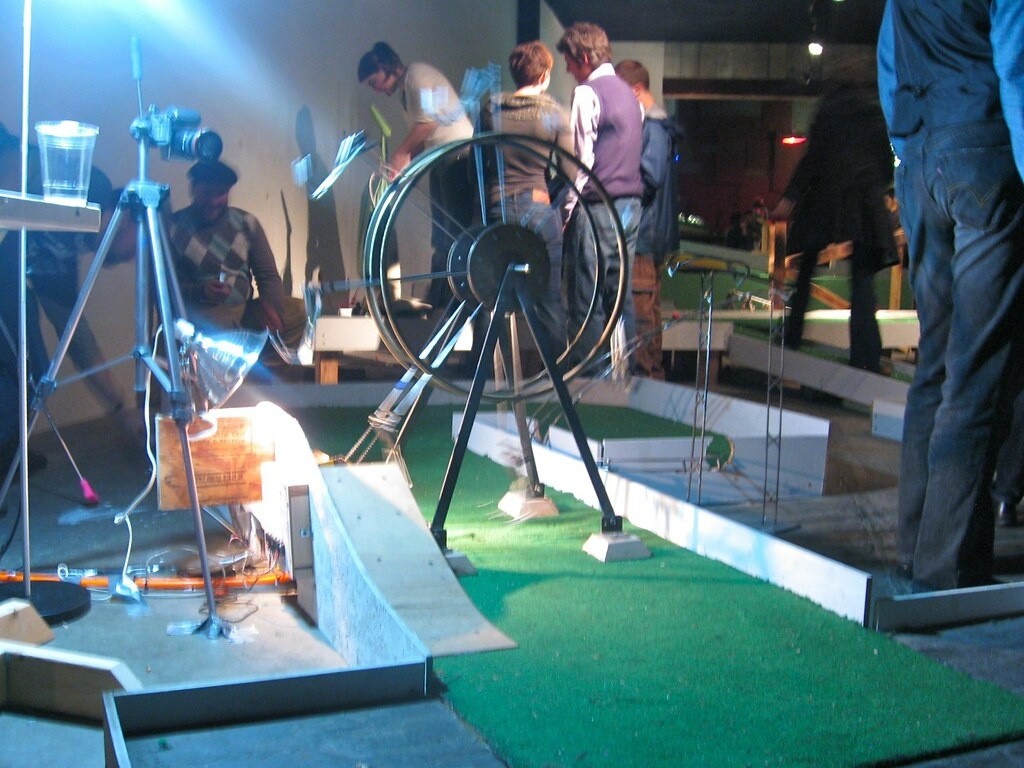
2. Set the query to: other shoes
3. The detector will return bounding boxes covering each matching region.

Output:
[995,499,1020,527]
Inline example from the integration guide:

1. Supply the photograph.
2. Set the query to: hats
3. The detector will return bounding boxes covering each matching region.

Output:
[187,156,240,186]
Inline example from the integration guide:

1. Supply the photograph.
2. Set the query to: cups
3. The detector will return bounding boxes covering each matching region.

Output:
[36,120,99,207]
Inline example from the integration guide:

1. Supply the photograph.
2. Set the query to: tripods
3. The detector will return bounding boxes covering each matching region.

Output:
[0,33,230,640]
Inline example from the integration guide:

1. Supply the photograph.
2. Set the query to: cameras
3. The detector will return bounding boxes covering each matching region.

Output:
[130,104,222,165]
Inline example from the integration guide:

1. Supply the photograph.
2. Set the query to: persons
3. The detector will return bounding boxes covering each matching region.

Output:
[0,121,137,487]
[614,60,684,382]
[770,67,894,366]
[877,1,1024,593]
[557,22,643,381]
[357,40,482,316]
[164,162,282,333]
[472,41,578,380]
[716,189,899,252]
